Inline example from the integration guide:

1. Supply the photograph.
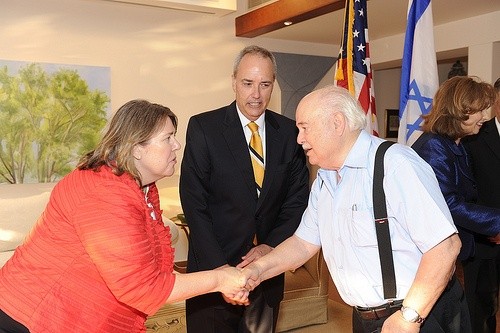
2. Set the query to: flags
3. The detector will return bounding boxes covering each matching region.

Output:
[335,0,380,136]
[397,0,439,147]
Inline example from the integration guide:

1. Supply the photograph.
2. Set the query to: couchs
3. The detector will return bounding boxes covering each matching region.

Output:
[276,243,330,333]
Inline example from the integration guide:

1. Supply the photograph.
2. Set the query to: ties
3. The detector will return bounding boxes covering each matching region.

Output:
[247,122,265,246]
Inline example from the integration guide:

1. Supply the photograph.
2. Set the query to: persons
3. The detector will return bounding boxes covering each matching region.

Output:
[177,43,310,333]
[460,72,500,332]
[1,98,253,333]
[406,71,500,332]
[230,84,475,333]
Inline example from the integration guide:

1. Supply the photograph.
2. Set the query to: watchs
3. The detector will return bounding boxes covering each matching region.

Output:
[398,305,427,325]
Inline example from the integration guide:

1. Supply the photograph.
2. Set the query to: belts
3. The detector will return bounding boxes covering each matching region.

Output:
[353,305,401,321]
